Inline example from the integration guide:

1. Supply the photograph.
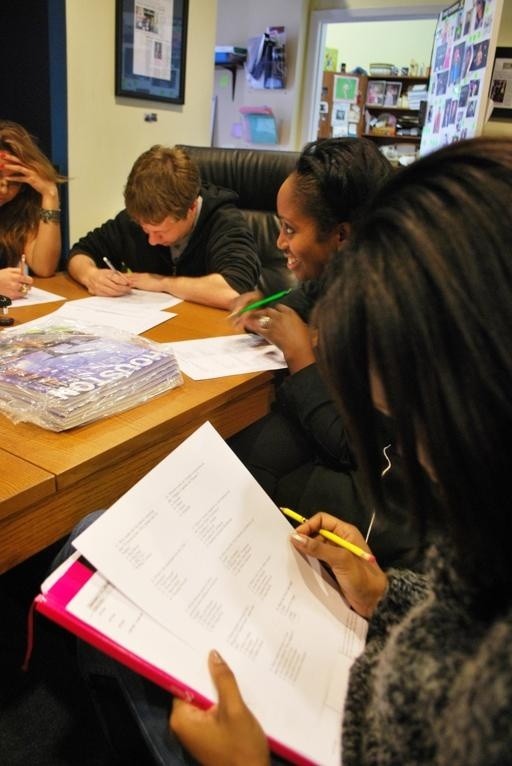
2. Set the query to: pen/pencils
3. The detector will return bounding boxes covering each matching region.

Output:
[103,257,120,276]
[225,287,293,320]
[21,254,25,276]
[279,506,376,564]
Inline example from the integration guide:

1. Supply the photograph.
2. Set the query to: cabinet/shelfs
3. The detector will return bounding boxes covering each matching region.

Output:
[314,67,433,173]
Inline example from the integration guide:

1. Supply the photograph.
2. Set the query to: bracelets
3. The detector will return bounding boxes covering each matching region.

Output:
[39,208,61,222]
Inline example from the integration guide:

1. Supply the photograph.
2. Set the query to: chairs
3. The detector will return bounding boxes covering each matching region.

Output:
[168,137,317,304]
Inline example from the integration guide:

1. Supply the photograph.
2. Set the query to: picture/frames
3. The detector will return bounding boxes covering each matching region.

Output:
[114,0,189,106]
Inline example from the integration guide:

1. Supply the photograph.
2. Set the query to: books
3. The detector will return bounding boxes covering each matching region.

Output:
[36,552,343,765]
[405,84,428,110]
[0,328,183,432]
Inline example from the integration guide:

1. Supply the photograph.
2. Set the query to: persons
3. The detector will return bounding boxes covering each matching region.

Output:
[0,121,68,300]
[473,0,486,29]
[51,134,510,765]
[242,138,431,572]
[470,50,484,70]
[66,146,263,310]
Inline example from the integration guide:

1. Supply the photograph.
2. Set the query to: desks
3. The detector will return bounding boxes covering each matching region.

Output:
[0,258,323,594]
